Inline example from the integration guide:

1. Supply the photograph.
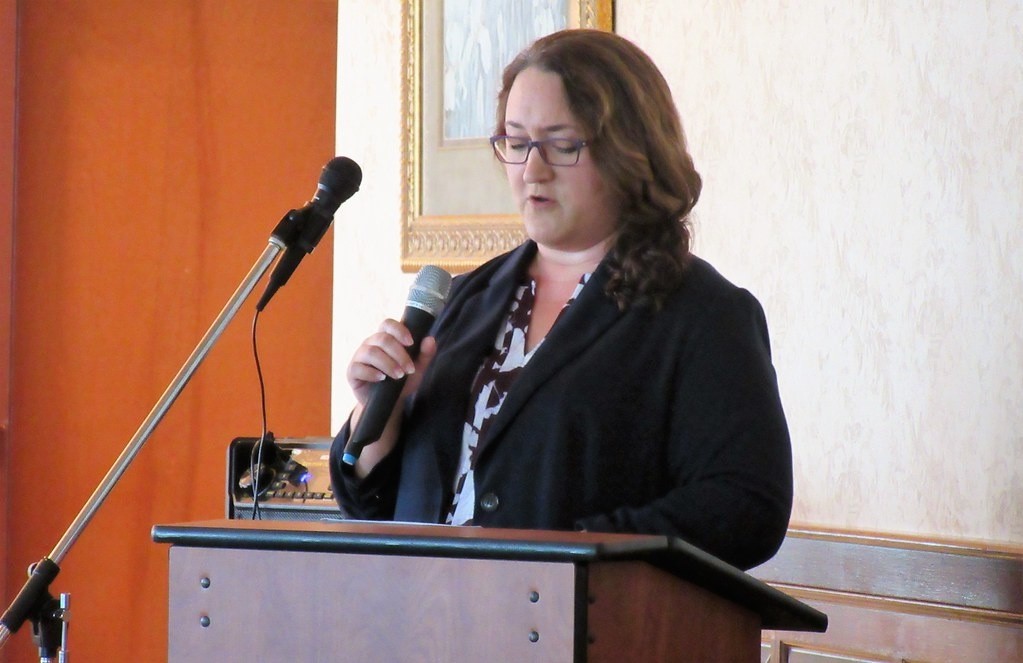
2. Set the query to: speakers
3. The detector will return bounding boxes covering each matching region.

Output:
[227,435,343,521]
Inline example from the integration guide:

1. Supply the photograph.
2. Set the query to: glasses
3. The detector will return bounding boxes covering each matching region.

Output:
[489,129,600,167]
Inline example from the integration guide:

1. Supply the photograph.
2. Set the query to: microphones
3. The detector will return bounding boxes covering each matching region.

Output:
[255,156,363,312]
[342,264,452,466]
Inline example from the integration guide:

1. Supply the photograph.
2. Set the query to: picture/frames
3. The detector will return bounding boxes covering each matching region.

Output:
[400,0,612,272]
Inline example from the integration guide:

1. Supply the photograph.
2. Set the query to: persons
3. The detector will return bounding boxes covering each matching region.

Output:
[330,29,792,574]
